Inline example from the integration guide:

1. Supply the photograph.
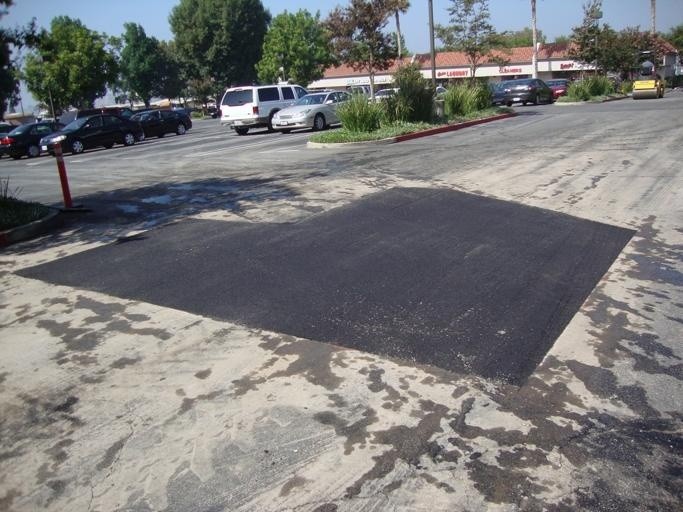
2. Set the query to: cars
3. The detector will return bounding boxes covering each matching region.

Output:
[0,103,221,160]
[486,78,572,108]
[270,85,448,132]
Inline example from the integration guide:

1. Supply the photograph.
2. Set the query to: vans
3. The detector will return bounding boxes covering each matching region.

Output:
[219,84,307,138]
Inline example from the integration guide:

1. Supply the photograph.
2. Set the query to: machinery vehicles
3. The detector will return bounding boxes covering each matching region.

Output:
[631,50,668,100]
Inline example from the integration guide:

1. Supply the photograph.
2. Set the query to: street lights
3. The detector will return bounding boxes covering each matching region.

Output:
[1,59,25,113]
[589,38,597,76]
